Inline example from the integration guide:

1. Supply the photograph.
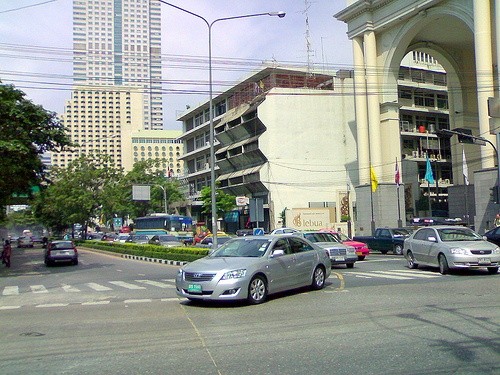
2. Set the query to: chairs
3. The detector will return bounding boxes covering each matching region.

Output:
[249,246,258,255]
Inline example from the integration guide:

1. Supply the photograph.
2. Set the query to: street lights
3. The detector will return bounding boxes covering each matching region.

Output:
[159,1,286,252]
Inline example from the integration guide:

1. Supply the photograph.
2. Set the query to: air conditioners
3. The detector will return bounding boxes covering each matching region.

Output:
[422,179,425,184]
[430,192,434,195]
[400,121,409,132]
[204,163,209,170]
[205,142,209,146]
[412,151,418,158]
[445,179,450,184]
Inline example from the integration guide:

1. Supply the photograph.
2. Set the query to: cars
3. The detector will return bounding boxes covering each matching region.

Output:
[41,241,79,266]
[320,230,370,261]
[403,217,500,274]
[175,233,331,305]
[17,236,34,248]
[196,232,235,250]
[124,235,150,245]
[291,231,358,268]
[481,226,500,245]
[268,227,300,238]
[73,223,129,244]
[148,234,184,247]
[31,235,43,243]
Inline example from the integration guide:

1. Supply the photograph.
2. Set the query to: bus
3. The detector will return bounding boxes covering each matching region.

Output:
[133,212,194,246]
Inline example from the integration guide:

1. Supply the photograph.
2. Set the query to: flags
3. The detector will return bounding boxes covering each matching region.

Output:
[371,166,377,193]
[346,172,350,192]
[425,154,434,184]
[395,162,399,187]
[463,148,470,186]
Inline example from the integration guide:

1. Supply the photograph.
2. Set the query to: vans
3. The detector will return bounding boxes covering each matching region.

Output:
[24,230,32,236]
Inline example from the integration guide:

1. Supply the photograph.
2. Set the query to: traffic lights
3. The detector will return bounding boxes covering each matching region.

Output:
[489,187,499,204]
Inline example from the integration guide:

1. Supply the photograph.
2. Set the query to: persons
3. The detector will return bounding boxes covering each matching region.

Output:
[494,213,500,228]
[3,240,11,267]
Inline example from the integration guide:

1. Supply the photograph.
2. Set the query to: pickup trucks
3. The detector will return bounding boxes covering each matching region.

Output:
[353,227,411,255]
